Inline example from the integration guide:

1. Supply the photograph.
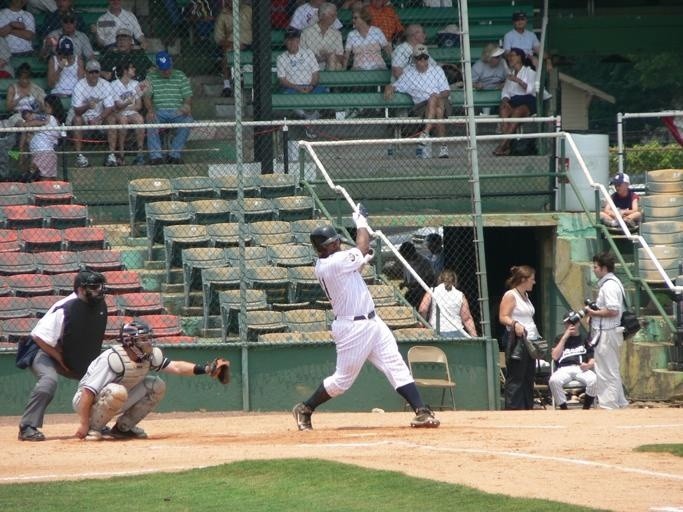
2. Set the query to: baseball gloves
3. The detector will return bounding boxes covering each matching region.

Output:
[211,357,230,384]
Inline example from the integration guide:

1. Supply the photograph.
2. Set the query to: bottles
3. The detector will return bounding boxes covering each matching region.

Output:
[415,141,424,159]
[387,144,393,159]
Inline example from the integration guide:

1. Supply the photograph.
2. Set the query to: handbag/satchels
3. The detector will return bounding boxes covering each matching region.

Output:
[525,338,549,359]
[622,310,640,341]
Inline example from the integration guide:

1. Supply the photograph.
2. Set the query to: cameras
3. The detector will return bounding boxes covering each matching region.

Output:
[569,298,597,325]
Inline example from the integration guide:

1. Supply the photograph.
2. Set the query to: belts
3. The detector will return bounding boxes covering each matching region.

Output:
[354,312,375,320]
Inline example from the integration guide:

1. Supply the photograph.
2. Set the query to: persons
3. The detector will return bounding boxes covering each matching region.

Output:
[1,1,196,184]
[471,12,553,156]
[394,226,627,411]
[151,0,424,138]
[17,269,105,440]
[599,172,643,228]
[292,202,442,430]
[386,43,452,158]
[71,320,230,439]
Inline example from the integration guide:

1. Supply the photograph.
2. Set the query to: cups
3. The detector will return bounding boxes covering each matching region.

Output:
[481,108,490,117]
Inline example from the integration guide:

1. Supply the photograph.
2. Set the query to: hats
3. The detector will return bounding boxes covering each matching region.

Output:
[84,60,101,71]
[284,26,301,38]
[116,29,132,36]
[609,173,630,186]
[157,51,171,69]
[562,310,576,324]
[413,44,429,57]
[482,44,505,59]
[59,10,76,55]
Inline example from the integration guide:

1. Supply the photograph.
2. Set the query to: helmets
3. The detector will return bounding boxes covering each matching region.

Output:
[122,321,154,362]
[73,270,108,318]
[309,225,341,249]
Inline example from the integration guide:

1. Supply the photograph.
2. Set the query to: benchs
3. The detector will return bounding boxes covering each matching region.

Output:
[1,1,553,170]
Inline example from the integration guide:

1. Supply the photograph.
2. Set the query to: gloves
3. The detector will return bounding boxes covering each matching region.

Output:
[352,203,368,229]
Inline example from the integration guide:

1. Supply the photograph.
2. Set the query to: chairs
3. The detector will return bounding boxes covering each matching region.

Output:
[402,344,458,412]
[0,171,434,349]
[632,167,682,326]
[498,345,598,410]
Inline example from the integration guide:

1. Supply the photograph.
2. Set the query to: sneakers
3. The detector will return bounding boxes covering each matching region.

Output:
[18,427,44,441]
[134,155,183,164]
[438,143,449,157]
[410,404,440,427]
[417,129,429,146]
[105,154,125,166]
[292,402,314,431]
[84,426,147,441]
[71,152,89,168]
